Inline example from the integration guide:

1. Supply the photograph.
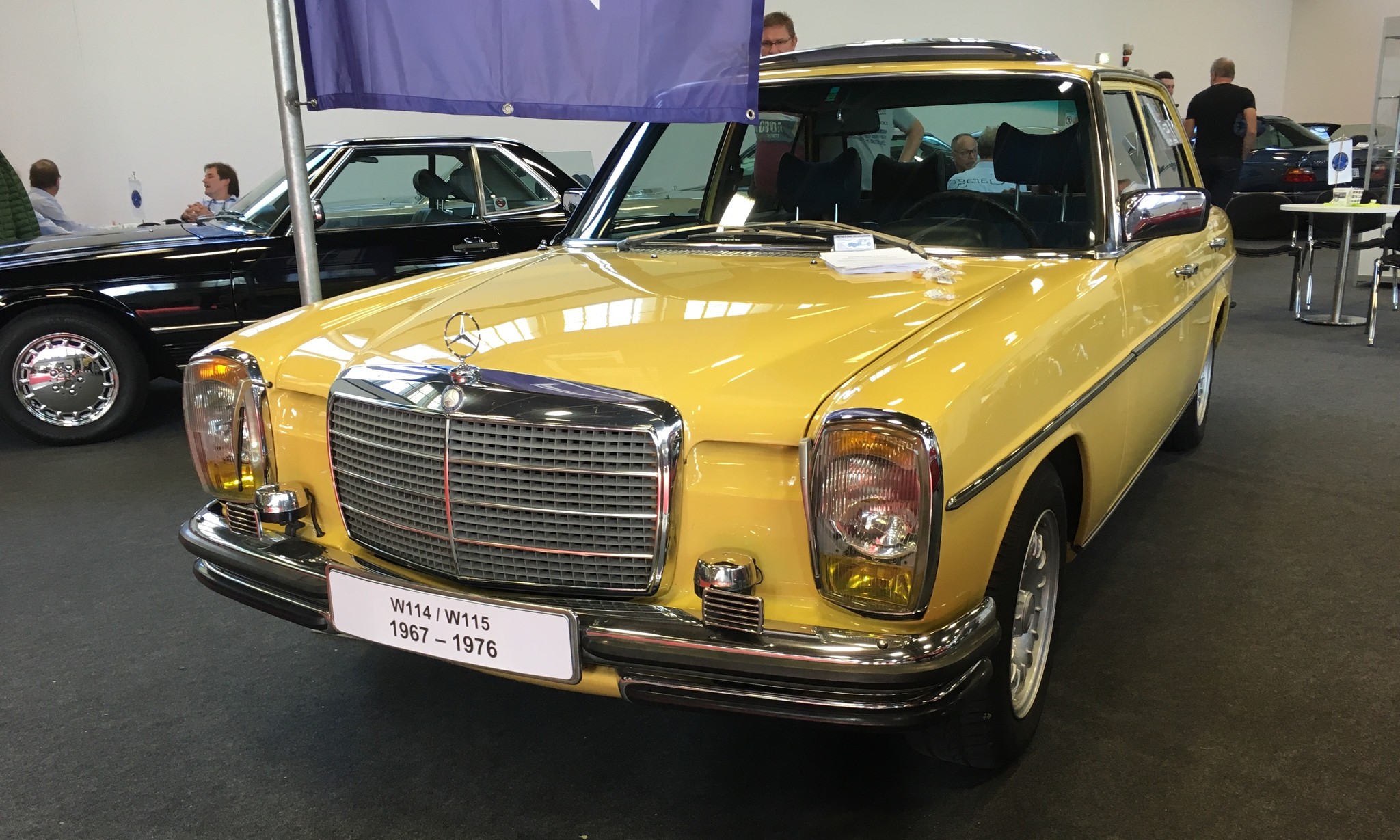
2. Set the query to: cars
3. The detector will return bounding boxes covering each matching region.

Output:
[675,122,1069,194]
[1184,113,1392,244]
[182,34,1235,777]
[0,135,698,441]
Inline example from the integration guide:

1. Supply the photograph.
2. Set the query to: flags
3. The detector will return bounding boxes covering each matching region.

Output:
[294,0,765,126]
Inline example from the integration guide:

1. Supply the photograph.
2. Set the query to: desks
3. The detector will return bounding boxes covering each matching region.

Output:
[1279,203,1400,325]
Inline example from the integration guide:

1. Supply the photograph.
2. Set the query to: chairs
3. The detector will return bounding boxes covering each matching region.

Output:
[777,147,862,222]
[1302,189,1397,311]
[1227,194,1302,319]
[993,123,1084,224]
[1364,211,1400,347]
[870,152,952,218]
[450,167,492,220]
[410,169,452,223]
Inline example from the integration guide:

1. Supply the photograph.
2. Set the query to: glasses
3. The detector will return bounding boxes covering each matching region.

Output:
[762,34,795,48]
[953,147,977,158]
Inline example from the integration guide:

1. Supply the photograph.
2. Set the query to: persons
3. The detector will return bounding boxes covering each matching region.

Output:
[27,159,116,236]
[181,162,240,224]
[754,11,1255,214]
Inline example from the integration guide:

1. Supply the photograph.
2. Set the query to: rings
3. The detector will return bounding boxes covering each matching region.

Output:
[192,211,195,213]
